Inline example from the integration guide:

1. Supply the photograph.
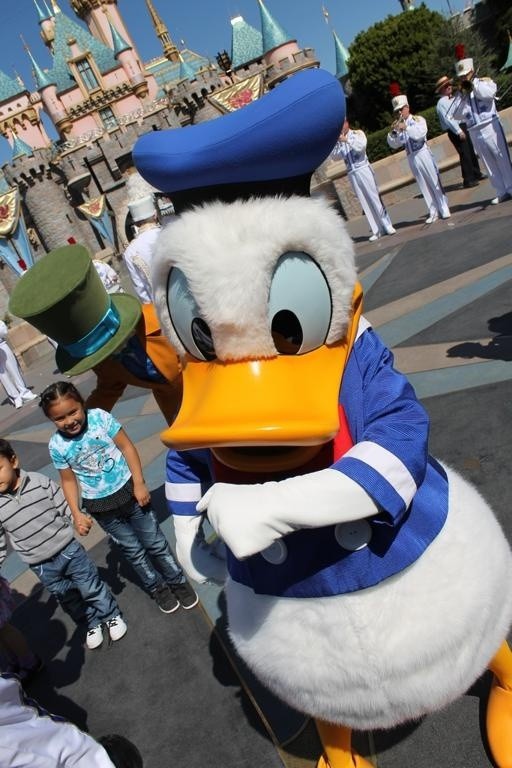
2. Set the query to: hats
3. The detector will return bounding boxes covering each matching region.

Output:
[455,57,475,78]
[392,94,409,113]
[435,76,454,93]
[127,195,156,225]
[8,243,144,377]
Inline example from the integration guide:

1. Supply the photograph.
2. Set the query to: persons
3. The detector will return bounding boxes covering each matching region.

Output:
[82,305,205,447]
[385,93,452,223]
[38,380,202,615]
[122,195,169,304]
[433,75,489,188]
[0,667,117,768]
[0,318,37,408]
[450,56,511,205]
[330,116,395,240]
[0,436,128,651]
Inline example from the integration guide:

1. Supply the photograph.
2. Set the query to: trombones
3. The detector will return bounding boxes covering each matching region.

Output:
[445,63,481,119]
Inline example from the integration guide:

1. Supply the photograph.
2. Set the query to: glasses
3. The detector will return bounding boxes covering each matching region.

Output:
[444,83,453,89]
[395,105,407,113]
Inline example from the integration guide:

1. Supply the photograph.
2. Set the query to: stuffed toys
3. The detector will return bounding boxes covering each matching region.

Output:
[131,70,512,768]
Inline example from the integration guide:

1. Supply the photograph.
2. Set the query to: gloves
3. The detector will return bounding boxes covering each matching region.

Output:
[461,80,474,93]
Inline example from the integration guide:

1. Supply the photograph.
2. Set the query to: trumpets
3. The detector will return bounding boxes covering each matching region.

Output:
[391,115,403,137]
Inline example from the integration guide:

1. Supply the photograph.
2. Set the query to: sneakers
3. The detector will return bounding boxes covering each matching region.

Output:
[85,622,104,650]
[151,584,180,615]
[463,172,508,205]
[15,399,24,409]
[105,614,128,642]
[22,393,39,404]
[369,209,451,242]
[170,580,200,610]
